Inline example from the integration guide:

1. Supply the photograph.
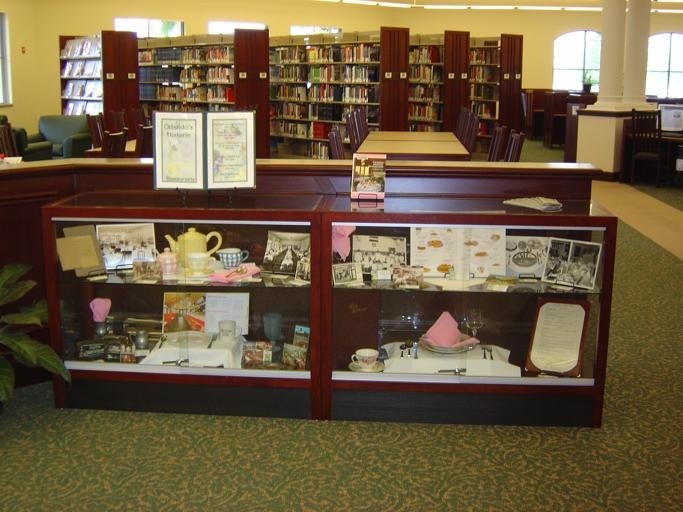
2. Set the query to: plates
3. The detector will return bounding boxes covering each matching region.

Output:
[181,270,215,278]
[347,360,383,373]
[418,334,476,355]
[165,331,209,349]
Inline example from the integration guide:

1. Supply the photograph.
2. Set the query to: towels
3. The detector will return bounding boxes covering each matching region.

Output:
[332,222,356,262]
[420,307,484,348]
[89,296,113,322]
[207,261,264,285]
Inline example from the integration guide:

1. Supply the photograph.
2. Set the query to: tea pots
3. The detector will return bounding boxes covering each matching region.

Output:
[164,226,222,269]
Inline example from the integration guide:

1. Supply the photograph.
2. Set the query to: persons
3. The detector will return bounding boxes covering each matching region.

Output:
[265,237,311,282]
[543,241,598,286]
[334,247,405,284]
[100,236,154,269]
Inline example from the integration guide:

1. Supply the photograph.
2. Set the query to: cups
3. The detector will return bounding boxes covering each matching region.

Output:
[215,247,249,270]
[351,348,379,371]
[188,252,215,274]
[153,247,177,274]
[127,329,149,350]
[261,312,282,342]
[217,319,242,344]
[87,297,111,323]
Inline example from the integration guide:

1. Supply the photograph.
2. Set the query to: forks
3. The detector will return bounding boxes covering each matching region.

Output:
[411,342,418,359]
[486,345,493,360]
[480,345,487,359]
[405,339,412,355]
[398,343,405,359]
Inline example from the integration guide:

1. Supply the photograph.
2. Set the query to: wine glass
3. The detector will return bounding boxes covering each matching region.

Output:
[463,304,484,338]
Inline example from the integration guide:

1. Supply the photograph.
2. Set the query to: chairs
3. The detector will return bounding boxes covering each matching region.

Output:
[630,107,673,188]
[0,107,87,164]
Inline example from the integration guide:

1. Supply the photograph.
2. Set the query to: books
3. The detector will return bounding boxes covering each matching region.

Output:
[138,34,234,123]
[408,34,444,132]
[269,32,380,159]
[148,107,259,192]
[60,34,102,114]
[470,38,498,135]
[349,151,388,201]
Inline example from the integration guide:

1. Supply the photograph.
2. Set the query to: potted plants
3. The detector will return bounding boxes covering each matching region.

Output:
[580,71,600,92]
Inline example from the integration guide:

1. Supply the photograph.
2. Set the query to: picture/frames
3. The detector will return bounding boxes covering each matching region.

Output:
[351,233,408,273]
[205,109,257,191]
[94,222,156,271]
[151,108,205,191]
[540,233,605,291]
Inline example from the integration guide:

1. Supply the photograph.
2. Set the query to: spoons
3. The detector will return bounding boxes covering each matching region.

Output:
[225,266,246,278]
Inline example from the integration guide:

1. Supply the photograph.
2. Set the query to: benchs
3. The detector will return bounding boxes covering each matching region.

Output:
[523,87,596,163]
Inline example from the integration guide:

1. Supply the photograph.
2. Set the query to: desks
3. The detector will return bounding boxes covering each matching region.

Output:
[620,117,682,188]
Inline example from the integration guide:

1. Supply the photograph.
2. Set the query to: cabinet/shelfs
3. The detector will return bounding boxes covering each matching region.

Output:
[323,193,622,427]
[38,193,331,422]
